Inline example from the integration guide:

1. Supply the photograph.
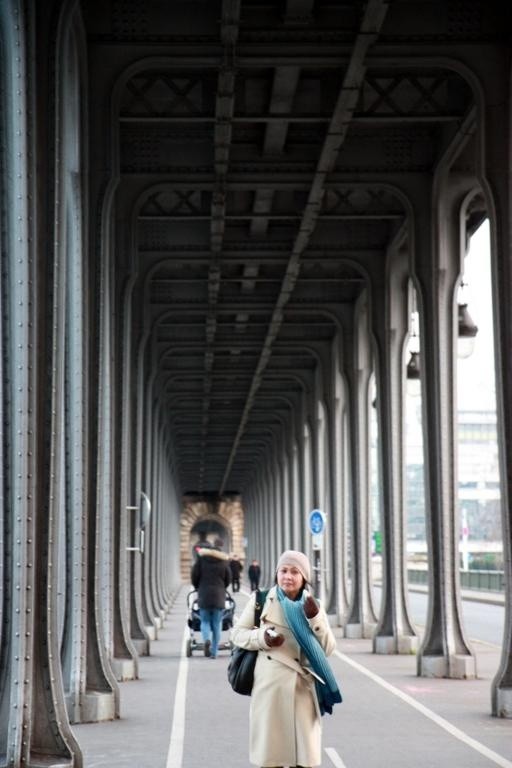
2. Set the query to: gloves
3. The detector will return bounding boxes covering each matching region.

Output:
[265,626,285,647]
[303,596,318,618]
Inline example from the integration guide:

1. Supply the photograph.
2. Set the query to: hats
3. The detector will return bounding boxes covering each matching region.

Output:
[276,550,311,584]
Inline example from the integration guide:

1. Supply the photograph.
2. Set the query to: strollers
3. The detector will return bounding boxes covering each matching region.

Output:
[184,588,236,658]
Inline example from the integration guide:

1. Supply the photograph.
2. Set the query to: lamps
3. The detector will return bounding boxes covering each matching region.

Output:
[458,281,478,358]
[407,313,422,397]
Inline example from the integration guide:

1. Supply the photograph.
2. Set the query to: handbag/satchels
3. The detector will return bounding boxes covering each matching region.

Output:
[227,646,258,695]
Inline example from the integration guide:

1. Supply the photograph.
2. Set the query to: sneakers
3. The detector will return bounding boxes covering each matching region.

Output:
[205,641,216,658]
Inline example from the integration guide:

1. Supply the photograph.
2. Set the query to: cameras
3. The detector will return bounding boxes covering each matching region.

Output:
[266,629,278,638]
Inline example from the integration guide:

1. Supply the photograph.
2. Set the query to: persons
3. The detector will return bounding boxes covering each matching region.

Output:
[229,555,243,592]
[230,550,336,767]
[249,559,261,591]
[191,546,233,659]
